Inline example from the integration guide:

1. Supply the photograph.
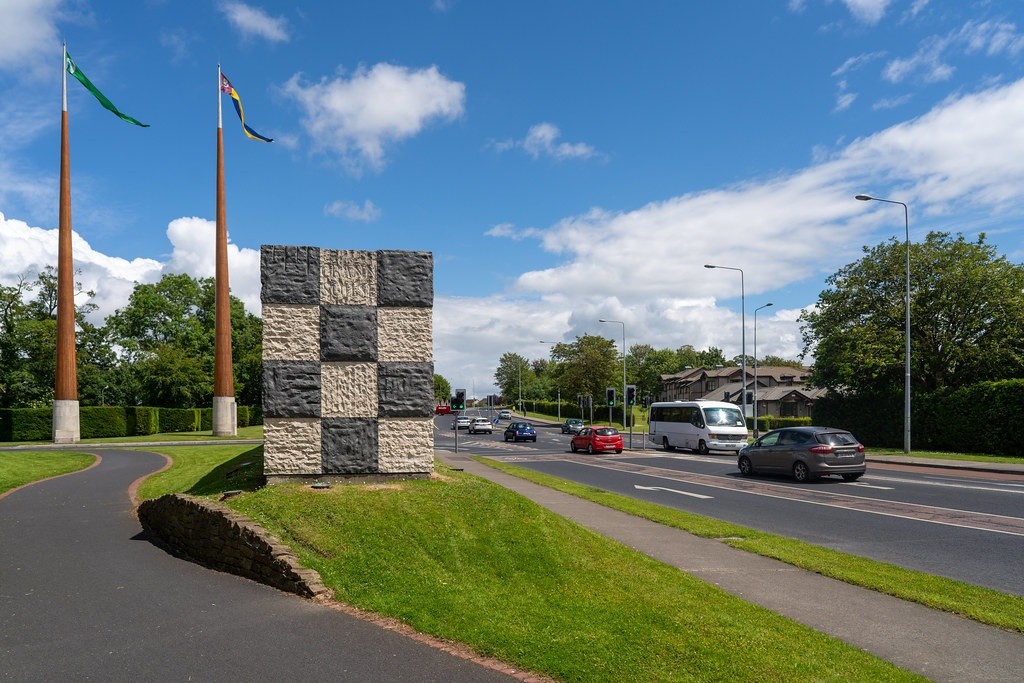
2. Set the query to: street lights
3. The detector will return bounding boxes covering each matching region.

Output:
[599,318,626,430]
[856,193,911,456]
[754,303,773,438]
[704,264,746,420]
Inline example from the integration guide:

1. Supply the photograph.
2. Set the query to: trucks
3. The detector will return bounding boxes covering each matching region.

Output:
[435,406,460,416]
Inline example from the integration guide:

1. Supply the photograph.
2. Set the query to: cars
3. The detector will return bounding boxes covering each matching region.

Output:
[452,416,470,430]
[571,427,623,455]
[498,411,512,420]
[468,418,493,435]
[504,422,537,443]
[737,426,867,485]
[561,419,584,435]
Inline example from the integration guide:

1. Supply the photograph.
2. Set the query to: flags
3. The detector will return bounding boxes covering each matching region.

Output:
[66,52,151,127]
[221,73,274,143]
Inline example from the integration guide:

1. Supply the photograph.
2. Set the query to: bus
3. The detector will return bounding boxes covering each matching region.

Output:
[648,401,749,455]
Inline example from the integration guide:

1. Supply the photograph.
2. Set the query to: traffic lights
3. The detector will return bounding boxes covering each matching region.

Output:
[606,388,616,408]
[642,397,647,409]
[450,397,457,411]
[626,385,636,406]
[455,389,466,411]
